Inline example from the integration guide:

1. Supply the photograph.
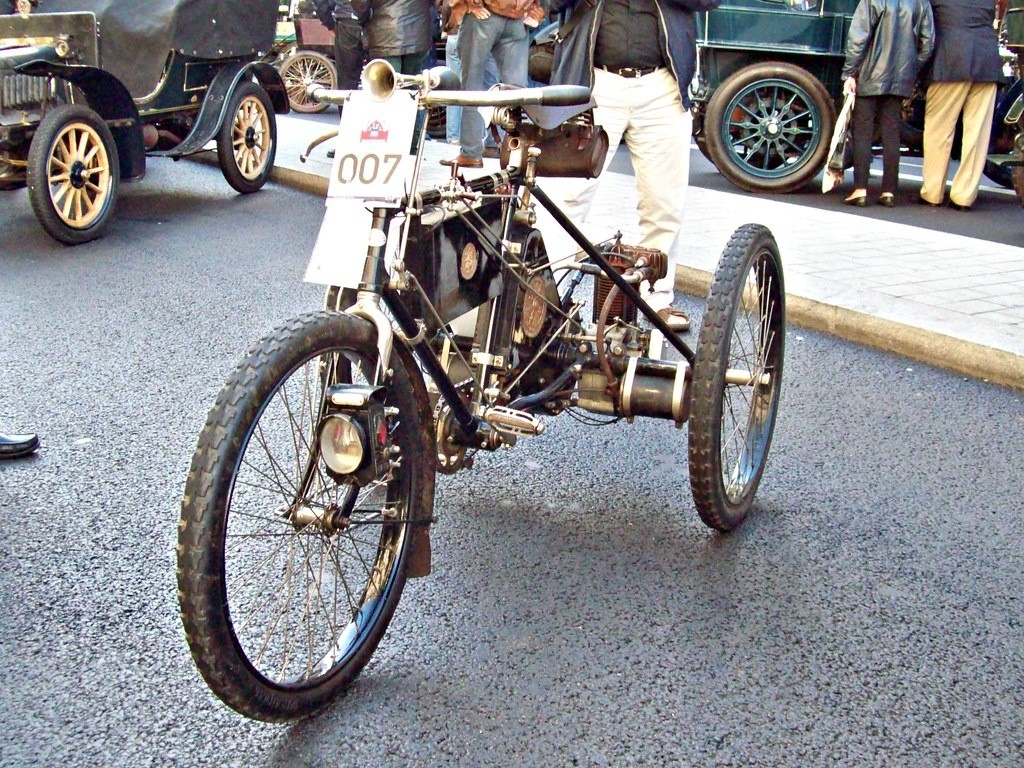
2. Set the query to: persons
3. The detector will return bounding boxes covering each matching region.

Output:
[548,0,724,330]
[309,0,432,158]
[908,0,1000,210]
[0,431,40,460]
[434,0,548,168]
[841,0,936,206]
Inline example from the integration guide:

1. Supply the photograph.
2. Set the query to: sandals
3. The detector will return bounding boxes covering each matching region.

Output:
[642,306,690,331]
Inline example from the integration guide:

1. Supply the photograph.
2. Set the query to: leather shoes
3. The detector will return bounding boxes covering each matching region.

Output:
[0,433,38,460]
[440,155,482,168]
[878,196,894,207]
[840,196,866,207]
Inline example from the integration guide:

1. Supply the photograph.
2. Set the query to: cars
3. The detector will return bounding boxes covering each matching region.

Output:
[0,1,291,244]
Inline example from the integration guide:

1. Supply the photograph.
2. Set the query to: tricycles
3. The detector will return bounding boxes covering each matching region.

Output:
[172,56,784,725]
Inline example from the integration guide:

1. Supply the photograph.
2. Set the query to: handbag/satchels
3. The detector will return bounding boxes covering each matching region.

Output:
[828,95,855,170]
[499,123,610,180]
[822,95,852,193]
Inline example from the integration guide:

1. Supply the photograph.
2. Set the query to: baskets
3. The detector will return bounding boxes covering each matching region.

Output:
[592,242,646,325]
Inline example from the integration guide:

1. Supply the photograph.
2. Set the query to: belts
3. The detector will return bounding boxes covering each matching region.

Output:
[595,65,654,77]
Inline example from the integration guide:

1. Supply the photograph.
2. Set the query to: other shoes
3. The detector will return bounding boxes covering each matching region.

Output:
[327,149,335,158]
[945,193,971,212]
[909,192,941,206]
